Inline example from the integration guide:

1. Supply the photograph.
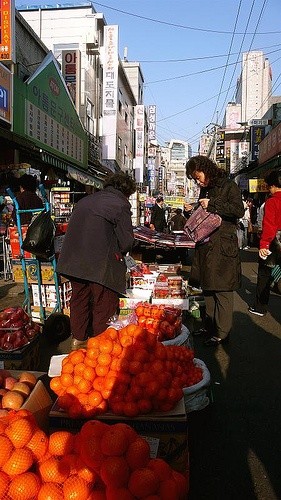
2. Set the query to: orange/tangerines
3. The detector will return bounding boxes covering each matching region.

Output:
[134,302,183,340]
[49,326,203,417]
[0,411,185,500]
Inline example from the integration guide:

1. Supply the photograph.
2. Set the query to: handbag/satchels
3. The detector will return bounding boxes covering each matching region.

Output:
[183,203,222,243]
[248,222,259,233]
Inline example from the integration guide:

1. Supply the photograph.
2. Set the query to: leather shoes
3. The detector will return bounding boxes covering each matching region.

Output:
[70,336,90,351]
[202,335,229,347]
[191,327,209,336]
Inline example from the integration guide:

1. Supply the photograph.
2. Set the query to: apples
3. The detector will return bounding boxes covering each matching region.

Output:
[0,306,31,329]
[1,323,40,351]
[0,370,36,409]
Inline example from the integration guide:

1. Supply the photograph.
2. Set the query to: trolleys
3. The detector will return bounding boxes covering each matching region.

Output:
[6,185,74,344]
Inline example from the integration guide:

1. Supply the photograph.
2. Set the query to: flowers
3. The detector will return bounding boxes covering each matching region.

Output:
[1,204,14,237]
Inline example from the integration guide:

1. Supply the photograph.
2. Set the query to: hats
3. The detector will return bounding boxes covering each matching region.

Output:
[156,196,162,203]
[265,171,280,188]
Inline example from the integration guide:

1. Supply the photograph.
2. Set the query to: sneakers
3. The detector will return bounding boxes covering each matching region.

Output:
[248,305,266,317]
[242,246,248,250]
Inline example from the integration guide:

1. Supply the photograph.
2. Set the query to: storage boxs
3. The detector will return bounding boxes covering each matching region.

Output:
[8,226,36,258]
[48,397,189,474]
[29,282,73,308]
[0,333,42,371]
[10,263,55,283]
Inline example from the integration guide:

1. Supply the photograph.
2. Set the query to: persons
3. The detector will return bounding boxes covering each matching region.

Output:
[237,223,244,249]
[56,170,137,347]
[248,171,281,318]
[185,156,244,347]
[170,209,185,230]
[13,174,43,224]
[150,196,166,231]
[241,193,251,250]
